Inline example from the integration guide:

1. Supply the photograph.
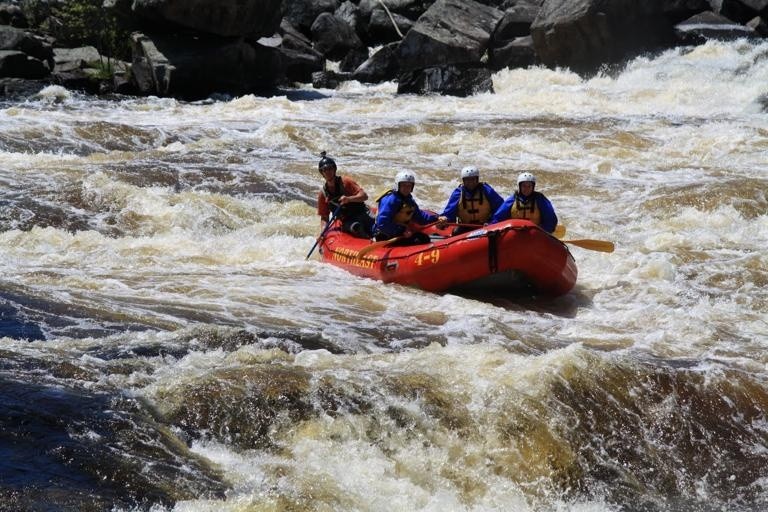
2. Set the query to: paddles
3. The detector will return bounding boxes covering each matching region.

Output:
[445,221,566,239]
[561,240,615,254]
[356,220,441,258]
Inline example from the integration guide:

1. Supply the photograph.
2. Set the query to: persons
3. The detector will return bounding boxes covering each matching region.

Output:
[314,150,375,247]
[435,163,505,238]
[482,171,559,236]
[368,168,450,249]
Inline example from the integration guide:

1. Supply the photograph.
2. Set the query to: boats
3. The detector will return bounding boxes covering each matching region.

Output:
[322,203,580,301]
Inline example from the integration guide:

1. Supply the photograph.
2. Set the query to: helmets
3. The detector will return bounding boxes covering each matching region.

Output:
[517,173,536,193]
[319,157,337,173]
[461,165,479,184]
[392,170,415,192]
